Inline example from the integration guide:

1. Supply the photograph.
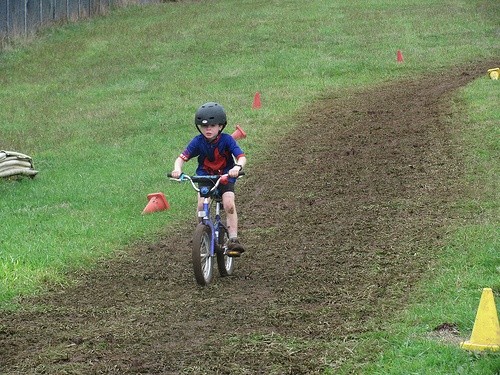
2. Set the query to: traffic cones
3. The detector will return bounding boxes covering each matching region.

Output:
[230,125,248,140]
[396,50,405,62]
[486,67,500,81]
[459,288,500,351]
[251,92,261,110]
[140,192,170,215]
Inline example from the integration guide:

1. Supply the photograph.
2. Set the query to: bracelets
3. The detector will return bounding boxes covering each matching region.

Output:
[235,163,243,171]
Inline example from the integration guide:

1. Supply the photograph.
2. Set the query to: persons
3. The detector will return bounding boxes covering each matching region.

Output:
[171,102,247,253]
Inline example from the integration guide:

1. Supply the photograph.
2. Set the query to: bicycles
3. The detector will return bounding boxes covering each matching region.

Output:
[166,171,247,287]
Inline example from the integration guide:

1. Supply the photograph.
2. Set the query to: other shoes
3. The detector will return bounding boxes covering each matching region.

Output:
[226,237,244,257]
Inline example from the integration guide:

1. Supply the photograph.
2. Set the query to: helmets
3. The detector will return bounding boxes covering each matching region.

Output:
[195,102,227,125]
